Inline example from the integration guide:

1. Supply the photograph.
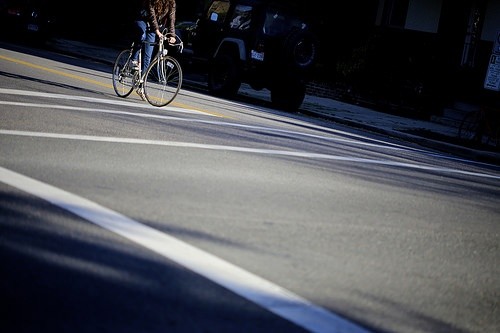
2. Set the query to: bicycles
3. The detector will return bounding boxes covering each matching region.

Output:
[111,40,183,107]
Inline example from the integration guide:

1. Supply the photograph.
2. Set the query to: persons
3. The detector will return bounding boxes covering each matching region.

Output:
[126,0,178,101]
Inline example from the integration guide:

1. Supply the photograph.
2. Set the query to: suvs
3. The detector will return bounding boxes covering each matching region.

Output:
[168,0,313,114]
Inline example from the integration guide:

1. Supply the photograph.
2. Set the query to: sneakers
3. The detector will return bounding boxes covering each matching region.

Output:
[129,60,139,70]
[137,79,144,83]
